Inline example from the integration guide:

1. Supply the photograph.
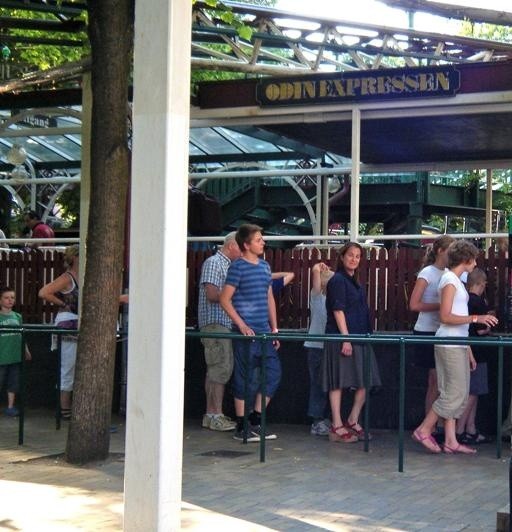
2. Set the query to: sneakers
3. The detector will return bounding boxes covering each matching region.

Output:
[310,419,332,436]
[234,423,277,442]
[4,407,20,415]
[202,413,236,431]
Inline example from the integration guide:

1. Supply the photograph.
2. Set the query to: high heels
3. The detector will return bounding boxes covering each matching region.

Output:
[345,422,374,441]
[329,424,358,443]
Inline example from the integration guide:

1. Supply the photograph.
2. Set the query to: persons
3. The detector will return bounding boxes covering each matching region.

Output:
[410,237,499,454]
[197,231,245,433]
[268,270,297,296]
[321,242,384,443]
[408,233,458,445]
[1,286,32,419]
[21,210,56,248]
[219,223,284,443]
[302,261,335,438]
[457,266,496,445]
[36,243,81,424]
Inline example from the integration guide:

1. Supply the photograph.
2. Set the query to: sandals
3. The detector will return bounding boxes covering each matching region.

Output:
[455,432,494,443]
[412,430,442,453]
[443,444,478,454]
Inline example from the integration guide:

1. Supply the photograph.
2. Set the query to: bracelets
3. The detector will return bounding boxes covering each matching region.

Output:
[471,313,479,323]
